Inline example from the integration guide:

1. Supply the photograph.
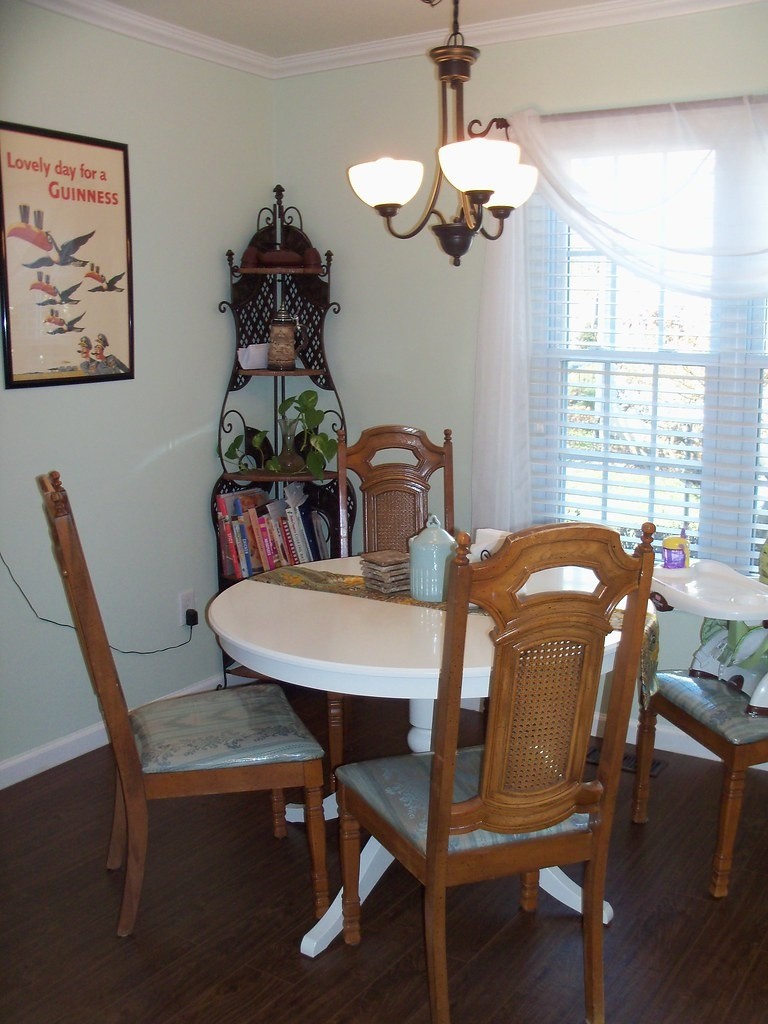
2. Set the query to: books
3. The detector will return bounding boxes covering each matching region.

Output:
[215,481,329,580]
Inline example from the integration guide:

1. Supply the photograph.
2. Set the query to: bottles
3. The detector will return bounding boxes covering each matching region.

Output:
[662,528,691,568]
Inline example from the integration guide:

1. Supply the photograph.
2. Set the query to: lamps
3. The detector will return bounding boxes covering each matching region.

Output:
[347,0,539,267]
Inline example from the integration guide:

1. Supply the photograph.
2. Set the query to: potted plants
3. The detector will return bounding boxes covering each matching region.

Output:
[217,390,337,481]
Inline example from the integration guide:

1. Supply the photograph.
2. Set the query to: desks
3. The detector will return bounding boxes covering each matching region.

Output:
[208,553,659,957]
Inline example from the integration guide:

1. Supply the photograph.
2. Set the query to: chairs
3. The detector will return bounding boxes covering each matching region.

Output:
[36,471,330,935]
[337,425,457,559]
[638,540,768,897]
[334,522,658,1024]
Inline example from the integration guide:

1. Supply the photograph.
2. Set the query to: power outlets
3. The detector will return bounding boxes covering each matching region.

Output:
[178,590,195,627]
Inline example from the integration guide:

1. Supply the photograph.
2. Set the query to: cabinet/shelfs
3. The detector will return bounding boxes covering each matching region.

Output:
[210,185,356,690]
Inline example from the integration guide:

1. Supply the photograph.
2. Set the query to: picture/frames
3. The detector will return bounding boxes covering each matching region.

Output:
[0,121,135,389]
